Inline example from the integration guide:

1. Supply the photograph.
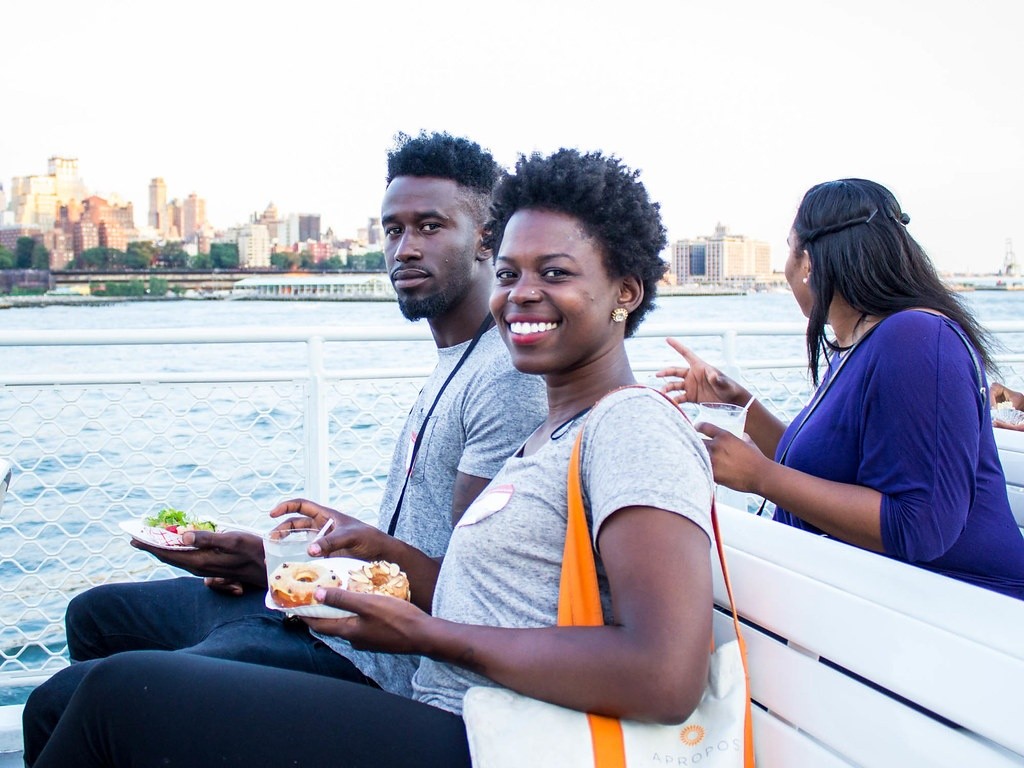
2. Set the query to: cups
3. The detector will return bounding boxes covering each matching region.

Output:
[262,528,325,592]
[698,402,748,439]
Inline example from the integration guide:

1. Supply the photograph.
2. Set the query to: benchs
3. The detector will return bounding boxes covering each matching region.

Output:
[986,418,1024,529]
[704,502,1024,768]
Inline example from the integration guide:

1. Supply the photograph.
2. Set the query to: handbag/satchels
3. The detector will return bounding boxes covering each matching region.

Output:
[461,386,756,767]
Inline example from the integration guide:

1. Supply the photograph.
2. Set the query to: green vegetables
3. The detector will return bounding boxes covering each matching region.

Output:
[146,511,217,532]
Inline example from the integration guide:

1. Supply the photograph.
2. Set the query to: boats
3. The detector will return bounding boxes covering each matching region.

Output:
[941,234,1024,293]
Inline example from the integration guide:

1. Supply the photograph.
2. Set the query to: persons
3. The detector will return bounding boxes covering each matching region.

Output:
[655,178,1024,730]
[988,381,1024,431]
[30,147,716,768]
[22,128,548,768]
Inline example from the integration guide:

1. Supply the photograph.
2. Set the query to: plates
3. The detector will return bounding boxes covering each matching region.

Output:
[264,557,371,619]
[118,519,202,551]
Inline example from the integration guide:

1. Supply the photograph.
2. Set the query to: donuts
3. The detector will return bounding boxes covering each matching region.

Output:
[270,560,409,608]
